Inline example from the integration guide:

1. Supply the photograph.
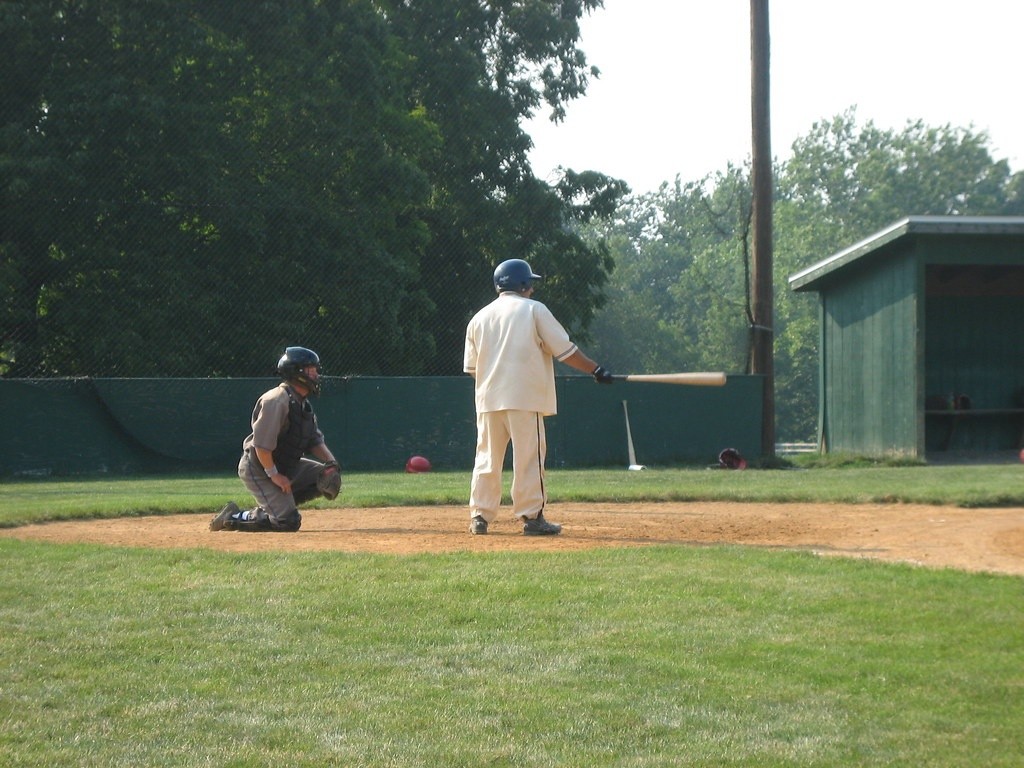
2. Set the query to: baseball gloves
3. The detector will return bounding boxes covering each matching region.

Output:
[317,461,343,500]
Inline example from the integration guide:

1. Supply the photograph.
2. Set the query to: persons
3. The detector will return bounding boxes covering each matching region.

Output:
[462,259,613,536]
[209,347,341,532]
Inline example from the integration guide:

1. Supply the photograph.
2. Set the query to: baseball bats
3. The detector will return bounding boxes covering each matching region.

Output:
[613,371,728,385]
[622,399,637,466]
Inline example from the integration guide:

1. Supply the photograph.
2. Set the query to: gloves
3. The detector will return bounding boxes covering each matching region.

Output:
[593,366,612,384]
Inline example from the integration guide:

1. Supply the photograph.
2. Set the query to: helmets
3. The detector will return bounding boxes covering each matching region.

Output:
[406,456,431,473]
[719,449,745,470]
[278,347,321,399]
[493,259,542,293]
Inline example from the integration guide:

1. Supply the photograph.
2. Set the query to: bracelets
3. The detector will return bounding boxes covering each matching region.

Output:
[265,464,278,478]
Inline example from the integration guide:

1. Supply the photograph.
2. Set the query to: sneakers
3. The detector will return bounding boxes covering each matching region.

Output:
[524,514,561,535]
[469,515,487,535]
[209,501,240,531]
